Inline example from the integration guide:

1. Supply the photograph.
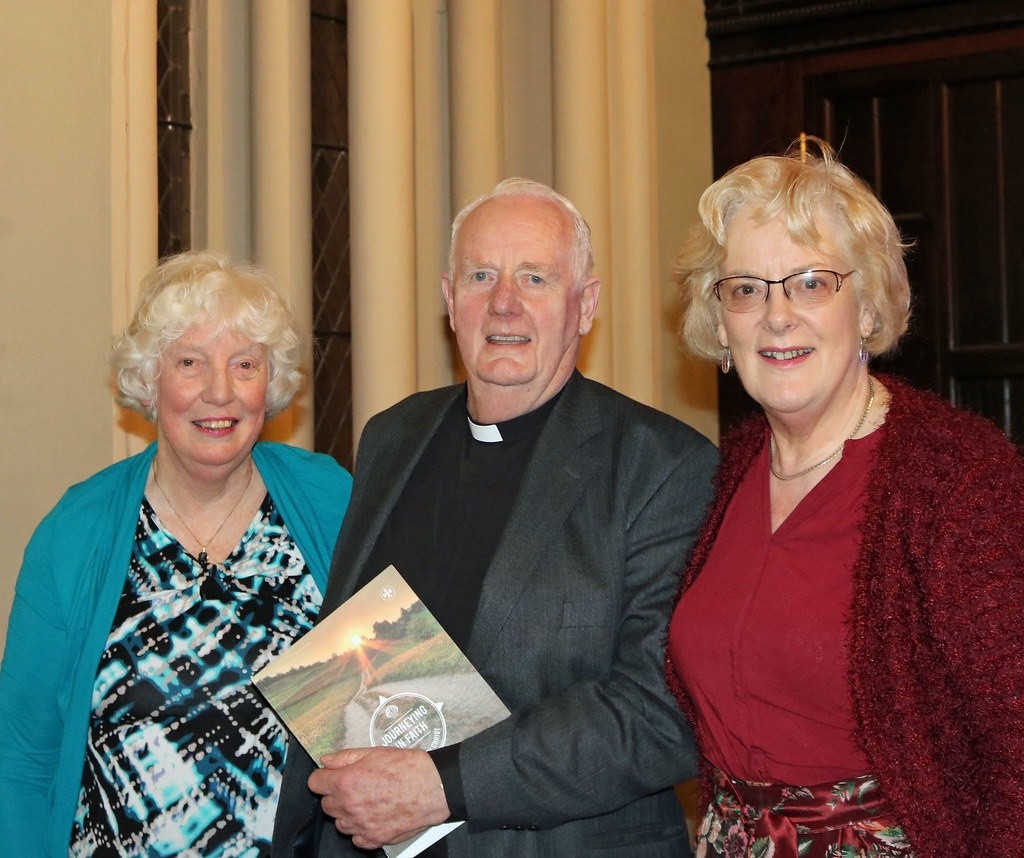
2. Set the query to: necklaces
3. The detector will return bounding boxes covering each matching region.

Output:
[152,458,253,564]
[768,377,874,481]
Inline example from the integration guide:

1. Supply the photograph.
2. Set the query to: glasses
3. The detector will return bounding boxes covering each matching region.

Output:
[712,268,856,314]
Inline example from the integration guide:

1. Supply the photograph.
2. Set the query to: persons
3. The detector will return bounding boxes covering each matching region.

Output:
[0,253,352,858]
[662,135,1024,856]
[269,178,720,858]
